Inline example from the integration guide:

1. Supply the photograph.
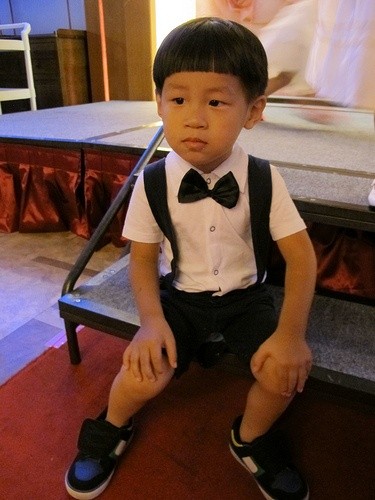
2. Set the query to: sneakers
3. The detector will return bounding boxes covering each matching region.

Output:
[228,415,309,500]
[64,406,134,500]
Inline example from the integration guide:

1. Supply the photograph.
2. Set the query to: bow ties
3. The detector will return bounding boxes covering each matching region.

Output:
[177,168,239,209]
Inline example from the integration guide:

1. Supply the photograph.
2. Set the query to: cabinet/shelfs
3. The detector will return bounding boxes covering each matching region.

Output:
[0,29,91,113]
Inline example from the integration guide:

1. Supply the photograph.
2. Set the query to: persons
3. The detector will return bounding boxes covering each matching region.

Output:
[64,17,317,500]
